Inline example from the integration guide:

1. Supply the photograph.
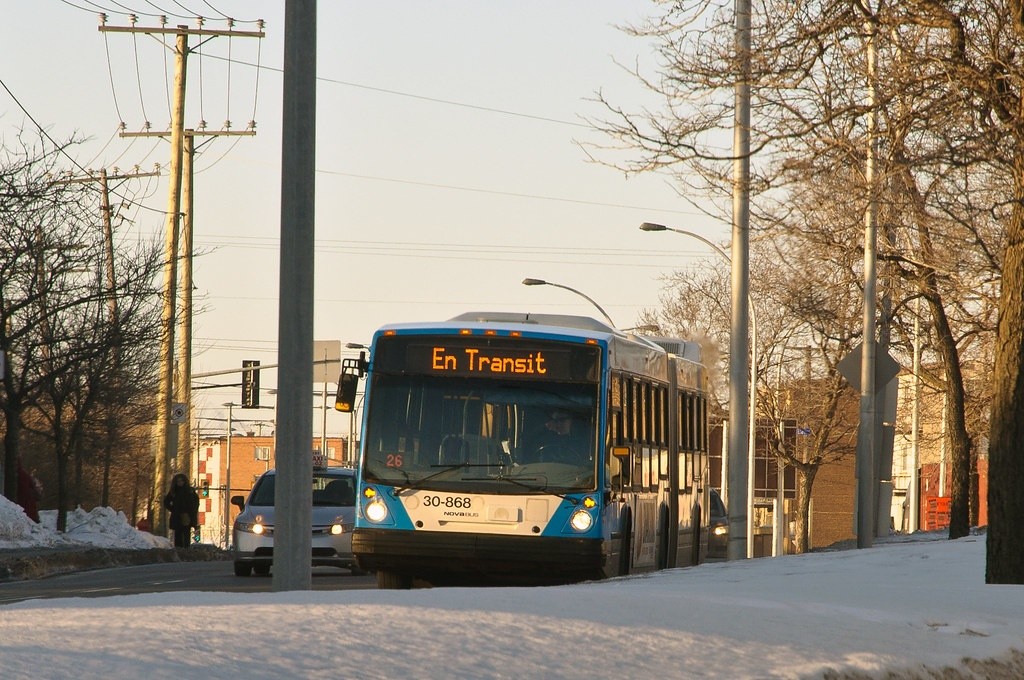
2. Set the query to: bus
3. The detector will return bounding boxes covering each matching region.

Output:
[334,312,712,590]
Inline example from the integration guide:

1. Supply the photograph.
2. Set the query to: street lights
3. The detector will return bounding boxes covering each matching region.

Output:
[638,221,757,557]
[222,403,230,549]
[267,388,327,489]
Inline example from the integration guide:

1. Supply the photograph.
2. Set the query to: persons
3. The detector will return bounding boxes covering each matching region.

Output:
[163,474,199,548]
[528,409,594,467]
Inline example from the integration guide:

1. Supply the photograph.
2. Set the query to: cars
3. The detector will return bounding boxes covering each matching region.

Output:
[230,467,368,578]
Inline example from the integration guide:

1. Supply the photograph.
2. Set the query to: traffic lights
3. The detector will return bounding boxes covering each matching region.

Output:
[201,479,209,498]
[192,525,200,542]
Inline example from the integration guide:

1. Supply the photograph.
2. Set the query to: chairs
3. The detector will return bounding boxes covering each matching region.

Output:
[439,433,469,466]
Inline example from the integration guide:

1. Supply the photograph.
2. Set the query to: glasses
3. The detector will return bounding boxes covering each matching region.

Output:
[551,415,570,421]
